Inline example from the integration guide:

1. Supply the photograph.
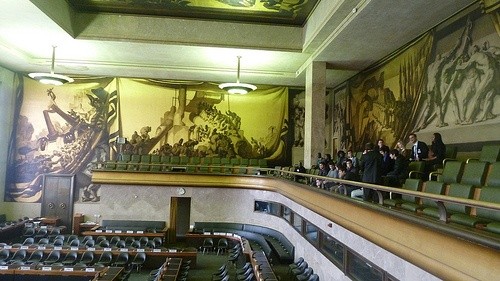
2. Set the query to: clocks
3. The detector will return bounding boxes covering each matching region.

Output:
[178,188,185,194]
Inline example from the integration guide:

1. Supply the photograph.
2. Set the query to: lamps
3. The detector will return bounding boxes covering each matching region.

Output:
[27,44,75,85]
[218,55,258,94]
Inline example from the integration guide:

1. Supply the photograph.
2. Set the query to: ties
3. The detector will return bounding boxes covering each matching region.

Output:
[414,145,416,159]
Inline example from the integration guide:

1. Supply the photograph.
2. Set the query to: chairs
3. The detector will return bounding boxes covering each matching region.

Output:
[95,139,500,240]
[1,215,323,281]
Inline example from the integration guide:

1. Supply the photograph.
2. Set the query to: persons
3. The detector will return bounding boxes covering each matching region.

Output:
[311,139,395,202]
[426,132,446,176]
[387,149,411,181]
[293,161,306,182]
[394,139,407,159]
[409,133,428,164]
